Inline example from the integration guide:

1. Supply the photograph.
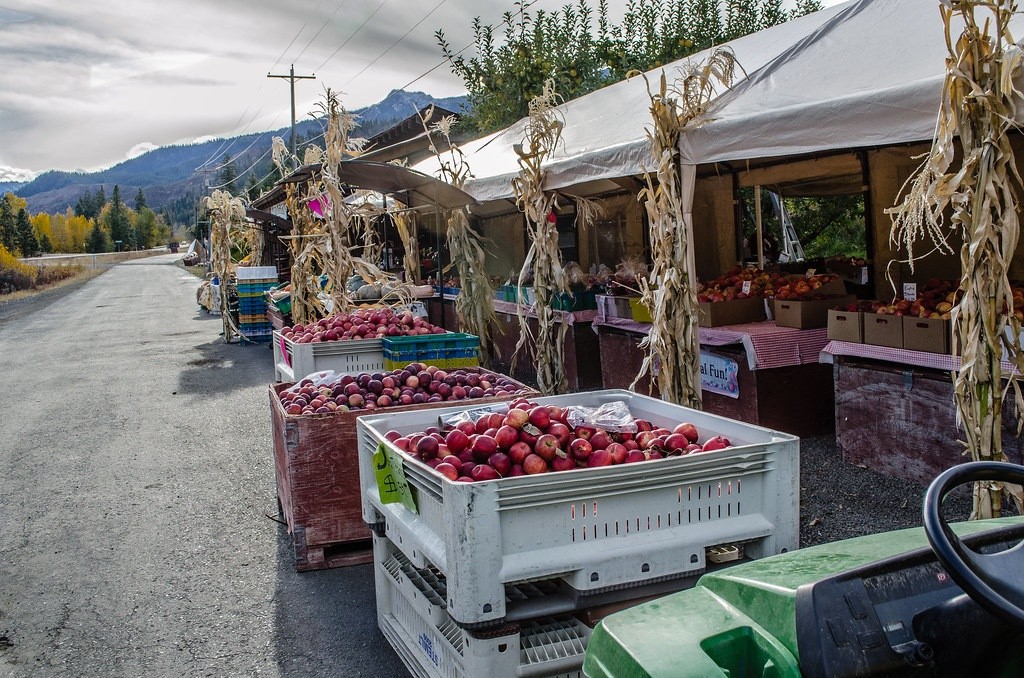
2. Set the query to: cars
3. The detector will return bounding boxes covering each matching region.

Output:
[182,240,187,246]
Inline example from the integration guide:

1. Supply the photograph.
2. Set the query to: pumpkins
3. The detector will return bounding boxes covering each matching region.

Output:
[347,273,434,300]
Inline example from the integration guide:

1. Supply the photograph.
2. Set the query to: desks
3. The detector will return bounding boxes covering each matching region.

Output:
[426,291,460,333]
[592,315,833,437]
[820,340,1024,516]
[490,299,600,393]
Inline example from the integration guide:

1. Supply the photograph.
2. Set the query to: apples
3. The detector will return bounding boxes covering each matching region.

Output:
[833,277,1024,326]
[695,265,830,304]
[281,308,446,343]
[280,362,527,415]
[832,255,866,267]
[383,397,729,484]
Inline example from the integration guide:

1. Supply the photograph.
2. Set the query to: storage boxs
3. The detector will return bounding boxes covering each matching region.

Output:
[493,284,652,323]
[774,294,858,329]
[272,331,384,384]
[777,275,847,296]
[234,266,278,344]
[269,367,542,572]
[696,301,766,327]
[357,388,802,678]
[827,306,952,354]
[382,333,480,371]
[267,281,293,315]
[435,287,462,295]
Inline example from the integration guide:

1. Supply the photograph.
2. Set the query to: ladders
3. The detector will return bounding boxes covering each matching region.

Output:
[769,192,806,262]
